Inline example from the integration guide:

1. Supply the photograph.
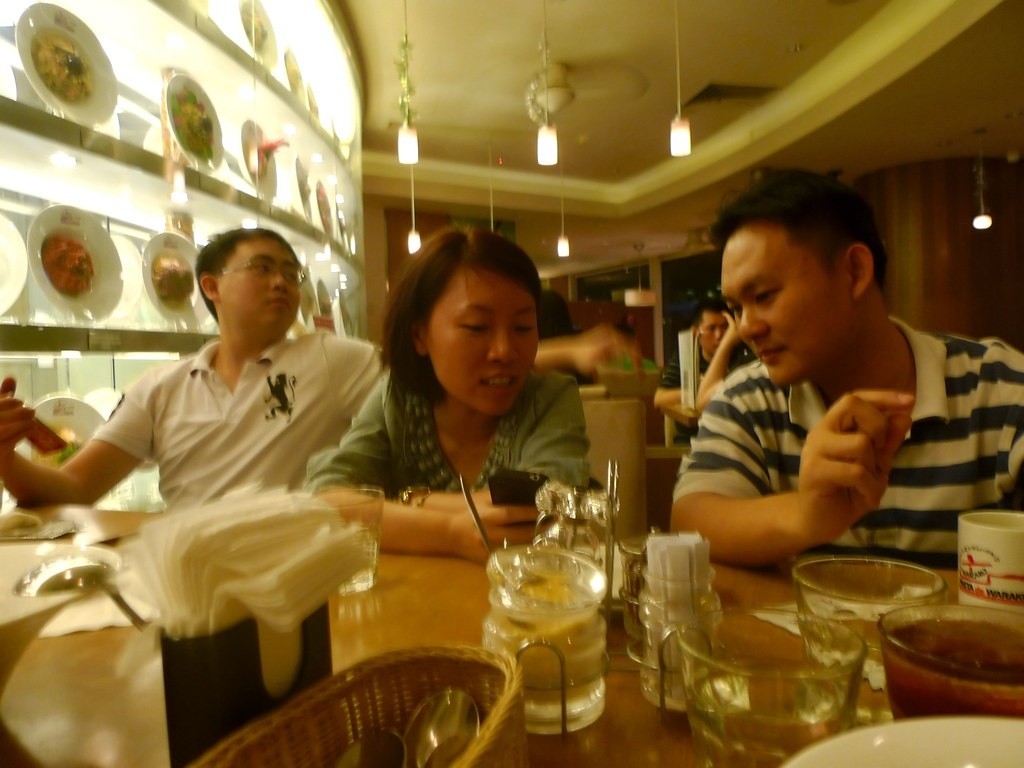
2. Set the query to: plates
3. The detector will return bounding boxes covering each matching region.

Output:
[0,0,364,465]
[1,542,122,639]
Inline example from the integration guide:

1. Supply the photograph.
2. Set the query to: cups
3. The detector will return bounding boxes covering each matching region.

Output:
[791,557,948,731]
[878,603,1024,722]
[776,716,1023,768]
[676,607,868,768]
[956,511,1023,612]
[480,547,609,739]
[313,485,385,595]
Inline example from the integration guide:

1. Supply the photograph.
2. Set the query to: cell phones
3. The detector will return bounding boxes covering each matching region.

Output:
[5,396,68,454]
[486,468,554,527]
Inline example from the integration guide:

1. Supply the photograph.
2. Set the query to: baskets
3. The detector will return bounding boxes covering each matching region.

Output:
[188,641,531,768]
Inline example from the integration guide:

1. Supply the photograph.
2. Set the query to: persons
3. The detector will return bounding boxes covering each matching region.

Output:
[670,168,1024,566]
[306,227,591,563]
[654,298,756,448]
[536,291,594,384]
[614,311,636,338]
[0,226,643,563]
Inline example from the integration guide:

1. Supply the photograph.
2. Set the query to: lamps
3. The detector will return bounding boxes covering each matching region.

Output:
[538,0,558,167]
[558,157,570,258]
[625,242,658,308]
[972,157,993,230]
[407,165,422,255]
[669,1,692,158]
[398,0,419,165]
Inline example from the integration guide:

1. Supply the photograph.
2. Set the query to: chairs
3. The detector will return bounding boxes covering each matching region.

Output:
[580,395,652,547]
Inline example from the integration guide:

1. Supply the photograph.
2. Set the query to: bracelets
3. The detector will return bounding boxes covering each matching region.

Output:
[401,486,433,508]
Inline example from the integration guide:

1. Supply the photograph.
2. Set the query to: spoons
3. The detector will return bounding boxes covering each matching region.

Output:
[11,553,148,634]
[331,689,478,768]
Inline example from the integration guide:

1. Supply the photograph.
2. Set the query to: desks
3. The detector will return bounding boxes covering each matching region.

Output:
[0,528,961,767]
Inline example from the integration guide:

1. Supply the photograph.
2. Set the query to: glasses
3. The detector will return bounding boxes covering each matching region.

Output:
[218,262,306,287]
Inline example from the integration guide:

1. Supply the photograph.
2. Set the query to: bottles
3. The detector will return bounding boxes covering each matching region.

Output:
[619,525,724,713]
